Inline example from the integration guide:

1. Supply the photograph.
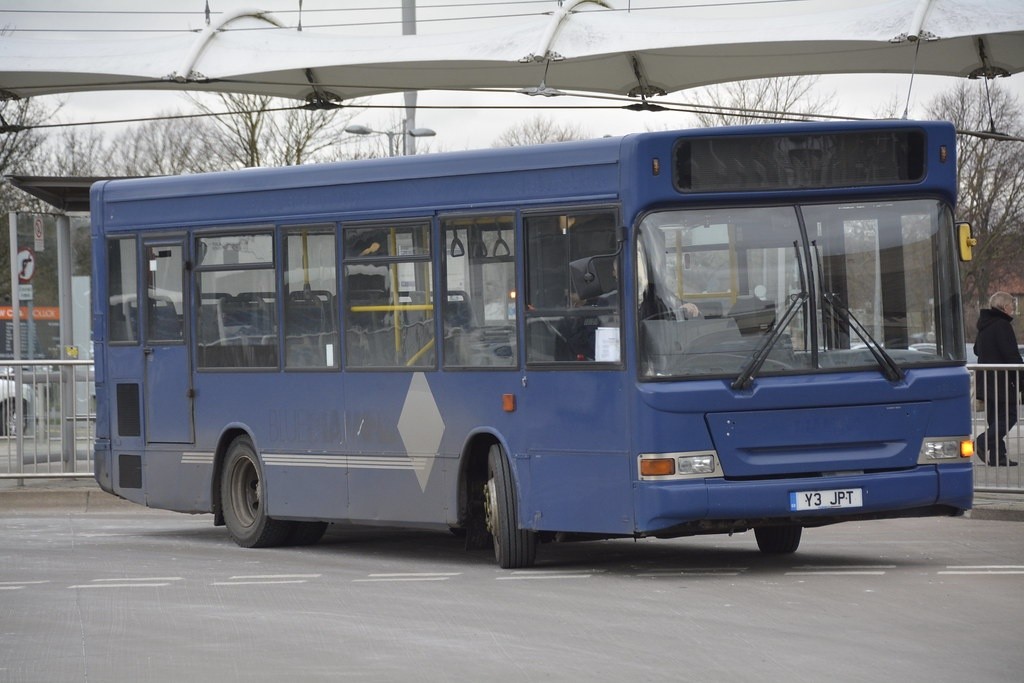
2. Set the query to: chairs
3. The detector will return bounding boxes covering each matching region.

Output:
[108,273,472,369]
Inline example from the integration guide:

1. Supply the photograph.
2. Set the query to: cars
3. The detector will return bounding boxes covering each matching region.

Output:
[0,362,42,440]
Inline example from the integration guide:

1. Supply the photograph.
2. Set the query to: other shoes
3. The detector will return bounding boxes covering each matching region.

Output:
[976,433,989,465]
[989,455,1017,466]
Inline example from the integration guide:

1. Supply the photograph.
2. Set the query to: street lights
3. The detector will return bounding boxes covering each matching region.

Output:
[342,124,437,158]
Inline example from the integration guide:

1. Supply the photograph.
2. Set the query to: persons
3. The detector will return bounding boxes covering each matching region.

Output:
[613,256,699,322]
[973,291,1024,467]
[553,291,602,362]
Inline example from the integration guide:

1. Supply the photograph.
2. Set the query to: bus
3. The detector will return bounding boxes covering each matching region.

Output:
[86,116,980,571]
